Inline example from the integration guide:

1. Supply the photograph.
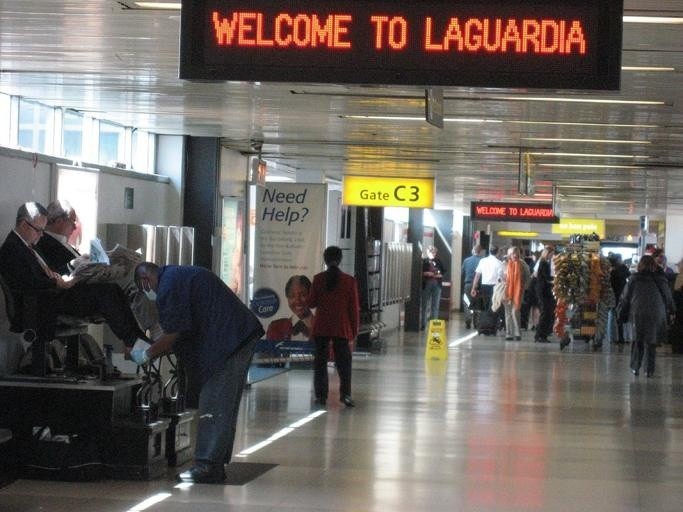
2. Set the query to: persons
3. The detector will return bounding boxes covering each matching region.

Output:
[130,261,263,481]
[309,245,361,408]
[421,244,443,328]
[616,254,669,376]
[1,200,157,356]
[266,272,314,343]
[462,244,682,357]
[38,199,162,339]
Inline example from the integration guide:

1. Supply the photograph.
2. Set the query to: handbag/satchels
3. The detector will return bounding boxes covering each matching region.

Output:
[492,285,507,311]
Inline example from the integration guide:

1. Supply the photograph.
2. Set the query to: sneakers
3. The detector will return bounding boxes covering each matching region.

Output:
[176,466,226,484]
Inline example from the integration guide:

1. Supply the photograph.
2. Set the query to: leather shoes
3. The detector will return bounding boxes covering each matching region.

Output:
[340,393,355,407]
[644,370,653,377]
[631,369,638,375]
[314,399,326,405]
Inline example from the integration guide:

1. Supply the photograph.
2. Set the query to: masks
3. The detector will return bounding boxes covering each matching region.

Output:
[141,280,156,301]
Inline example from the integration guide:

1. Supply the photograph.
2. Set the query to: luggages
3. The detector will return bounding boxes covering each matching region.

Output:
[478,307,498,336]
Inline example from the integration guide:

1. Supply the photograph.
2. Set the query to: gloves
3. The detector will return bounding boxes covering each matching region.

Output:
[129,349,147,365]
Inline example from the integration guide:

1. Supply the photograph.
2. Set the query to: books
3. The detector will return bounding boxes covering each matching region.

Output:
[105,241,143,278]
[73,237,110,283]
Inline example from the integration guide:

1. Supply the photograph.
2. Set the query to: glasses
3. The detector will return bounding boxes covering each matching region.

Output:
[23,219,44,232]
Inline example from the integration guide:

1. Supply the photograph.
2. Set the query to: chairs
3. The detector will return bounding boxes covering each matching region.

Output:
[0,277,87,381]
